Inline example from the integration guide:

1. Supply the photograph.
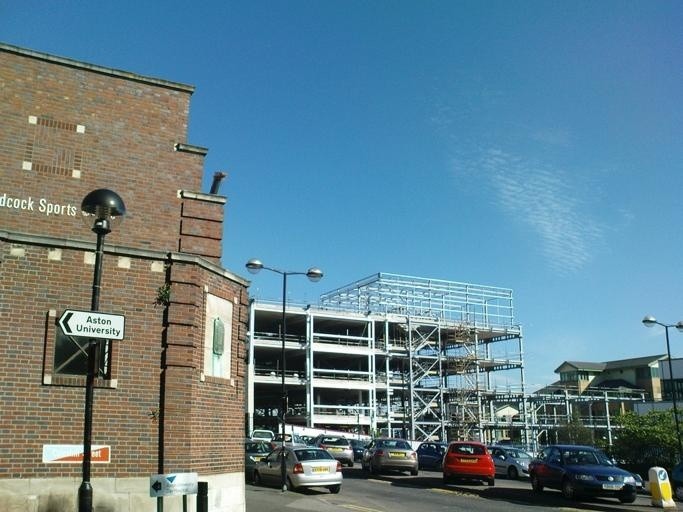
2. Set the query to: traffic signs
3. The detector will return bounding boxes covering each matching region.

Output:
[57,308,124,341]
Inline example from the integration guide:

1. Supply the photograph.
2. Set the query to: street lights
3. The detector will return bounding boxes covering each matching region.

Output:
[79,187,127,512]
[642,316,683,460]
[245,258,323,492]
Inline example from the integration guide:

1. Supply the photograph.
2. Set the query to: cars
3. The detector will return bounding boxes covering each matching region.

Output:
[253,446,343,494]
[416,441,447,472]
[440,441,496,486]
[486,445,534,480]
[309,435,355,467]
[528,444,637,504]
[246,441,274,482]
[355,438,419,476]
[350,439,372,463]
[292,434,315,445]
[669,459,683,501]
[629,471,645,494]
[270,433,306,451]
[247,430,276,452]
